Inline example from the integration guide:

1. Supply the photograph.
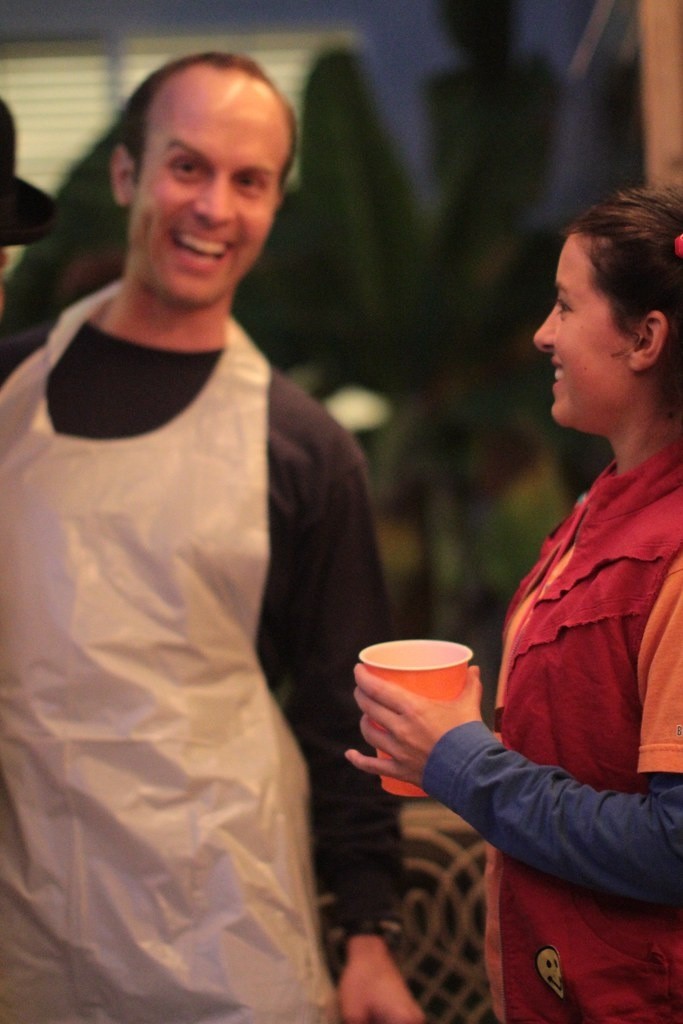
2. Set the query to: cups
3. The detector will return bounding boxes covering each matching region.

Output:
[359,639,475,798]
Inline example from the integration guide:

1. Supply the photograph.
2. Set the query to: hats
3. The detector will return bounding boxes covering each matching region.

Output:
[0,92,61,247]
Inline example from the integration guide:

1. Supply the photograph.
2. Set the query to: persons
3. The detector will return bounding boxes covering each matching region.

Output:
[2,51,425,1024]
[345,186,683,1024]
